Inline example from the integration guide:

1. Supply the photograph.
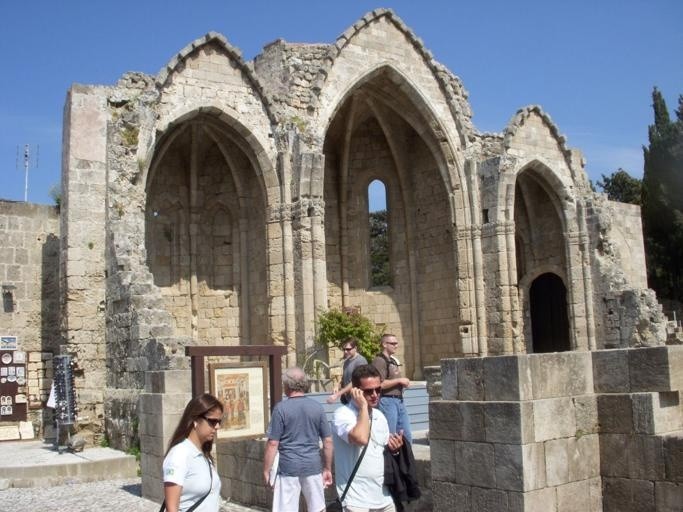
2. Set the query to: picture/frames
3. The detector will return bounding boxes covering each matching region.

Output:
[207,360,269,444]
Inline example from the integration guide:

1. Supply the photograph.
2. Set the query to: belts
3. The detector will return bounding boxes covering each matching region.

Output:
[382,394,403,400]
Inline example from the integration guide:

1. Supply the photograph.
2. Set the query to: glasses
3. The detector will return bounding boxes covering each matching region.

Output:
[358,386,384,397]
[199,416,223,426]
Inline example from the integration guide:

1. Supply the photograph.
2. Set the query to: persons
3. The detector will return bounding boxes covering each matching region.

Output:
[331,362,406,511]
[160,392,229,511]
[324,336,366,406]
[261,366,335,511]
[366,332,412,442]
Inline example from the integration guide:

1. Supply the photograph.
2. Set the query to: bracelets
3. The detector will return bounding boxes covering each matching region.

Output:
[391,452,399,456]
